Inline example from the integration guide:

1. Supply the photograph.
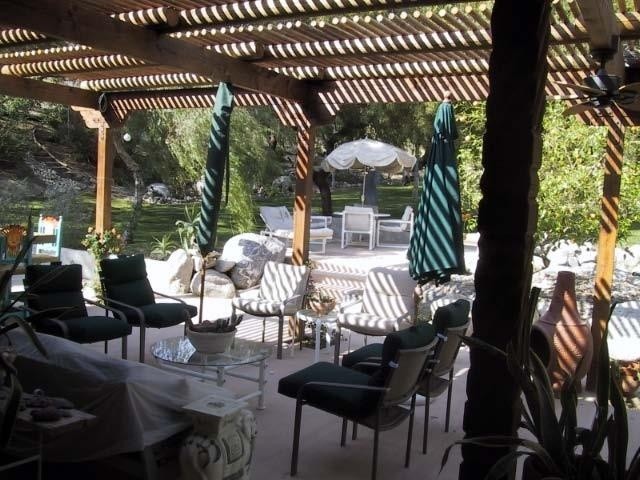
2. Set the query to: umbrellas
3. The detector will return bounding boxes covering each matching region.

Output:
[407,89,469,327]
[320,135,417,207]
[194,79,236,326]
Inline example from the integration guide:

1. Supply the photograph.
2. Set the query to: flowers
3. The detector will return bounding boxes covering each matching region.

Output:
[82,226,125,262]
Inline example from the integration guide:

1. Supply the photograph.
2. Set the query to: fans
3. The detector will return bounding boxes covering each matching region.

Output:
[553,44,640,117]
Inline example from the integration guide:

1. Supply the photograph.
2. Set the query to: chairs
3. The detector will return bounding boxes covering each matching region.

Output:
[332,267,419,367]
[230,260,311,361]
[99,252,198,365]
[0,225,34,308]
[29,216,64,267]
[257,201,415,259]
[341,298,470,454]
[278,324,436,478]
[23,265,132,359]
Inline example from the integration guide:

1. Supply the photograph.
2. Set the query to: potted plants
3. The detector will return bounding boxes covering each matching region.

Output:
[182,303,244,352]
[438,278,640,479]
[306,289,337,314]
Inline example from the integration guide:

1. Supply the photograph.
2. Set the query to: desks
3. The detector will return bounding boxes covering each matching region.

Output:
[150,337,273,412]
[2,231,57,245]
[295,310,339,365]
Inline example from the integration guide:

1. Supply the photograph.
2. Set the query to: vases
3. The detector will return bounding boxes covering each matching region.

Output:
[92,260,103,292]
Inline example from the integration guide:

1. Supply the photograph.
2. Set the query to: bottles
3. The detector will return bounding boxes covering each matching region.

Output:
[537,269,595,401]
